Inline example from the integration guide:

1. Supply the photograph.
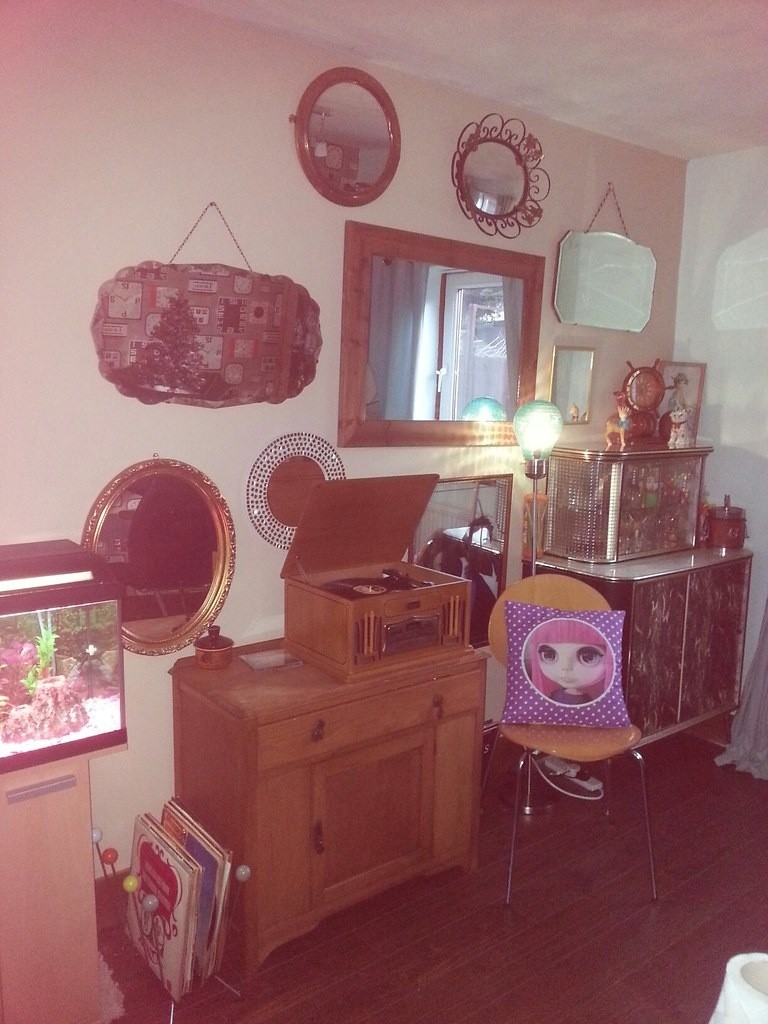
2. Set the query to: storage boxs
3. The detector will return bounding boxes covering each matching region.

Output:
[543,445,713,563]
[0,539,129,774]
[275,471,473,685]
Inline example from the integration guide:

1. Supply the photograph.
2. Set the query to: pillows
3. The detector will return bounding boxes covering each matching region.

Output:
[500,599,631,730]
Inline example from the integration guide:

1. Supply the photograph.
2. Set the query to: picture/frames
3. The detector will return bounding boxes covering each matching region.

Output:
[659,361,706,442]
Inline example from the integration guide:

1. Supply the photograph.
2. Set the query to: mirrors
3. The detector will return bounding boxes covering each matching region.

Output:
[549,344,596,425]
[294,66,401,207]
[451,112,551,236]
[337,221,545,449]
[80,457,237,658]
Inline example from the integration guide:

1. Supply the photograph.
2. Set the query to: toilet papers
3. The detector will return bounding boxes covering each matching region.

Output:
[708,951,768,1024]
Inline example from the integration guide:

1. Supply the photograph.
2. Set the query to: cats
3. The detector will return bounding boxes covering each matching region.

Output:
[668,405,696,450]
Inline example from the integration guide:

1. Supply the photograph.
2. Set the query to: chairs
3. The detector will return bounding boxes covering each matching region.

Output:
[106,480,193,614]
[486,574,660,905]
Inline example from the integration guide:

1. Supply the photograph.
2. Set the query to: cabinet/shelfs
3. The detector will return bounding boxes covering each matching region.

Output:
[169,637,492,1000]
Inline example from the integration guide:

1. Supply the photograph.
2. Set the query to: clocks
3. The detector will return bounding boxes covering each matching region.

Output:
[324,144,344,170]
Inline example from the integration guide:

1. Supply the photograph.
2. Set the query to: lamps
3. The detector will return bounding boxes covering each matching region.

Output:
[459,394,508,422]
[514,400,564,575]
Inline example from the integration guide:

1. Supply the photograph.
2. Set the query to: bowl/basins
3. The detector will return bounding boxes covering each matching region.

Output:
[192,625,235,670]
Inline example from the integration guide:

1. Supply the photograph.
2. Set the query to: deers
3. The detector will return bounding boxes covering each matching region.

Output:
[604,406,632,450]
